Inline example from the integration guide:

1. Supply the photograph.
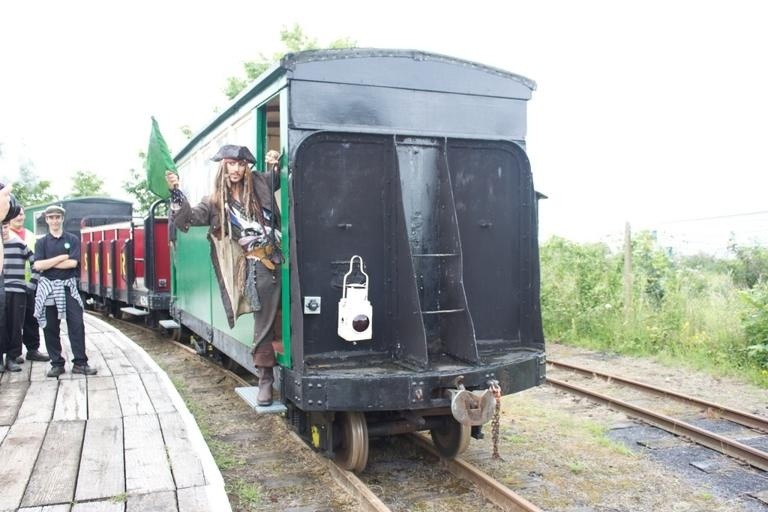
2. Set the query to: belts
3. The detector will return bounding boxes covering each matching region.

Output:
[242,245,275,257]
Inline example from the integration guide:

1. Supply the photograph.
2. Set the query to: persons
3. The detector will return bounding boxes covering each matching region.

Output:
[0,219,39,372]
[9,205,50,363]
[163,144,281,407]
[0,183,14,273]
[33,205,99,377]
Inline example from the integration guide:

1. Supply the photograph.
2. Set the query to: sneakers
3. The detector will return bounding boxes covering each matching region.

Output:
[25,349,51,362]
[5,357,23,372]
[46,363,66,378]
[0,352,4,373]
[14,355,25,364]
[71,363,99,376]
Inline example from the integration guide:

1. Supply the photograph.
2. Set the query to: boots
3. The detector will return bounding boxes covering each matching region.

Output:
[255,363,276,405]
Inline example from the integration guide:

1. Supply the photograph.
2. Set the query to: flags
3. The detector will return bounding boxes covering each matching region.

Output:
[143,115,179,201]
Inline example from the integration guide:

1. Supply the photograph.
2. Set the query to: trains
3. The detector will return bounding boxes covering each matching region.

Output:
[23,47,547,475]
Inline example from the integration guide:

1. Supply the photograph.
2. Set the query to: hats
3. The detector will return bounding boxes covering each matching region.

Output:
[44,205,66,217]
[208,144,256,164]
